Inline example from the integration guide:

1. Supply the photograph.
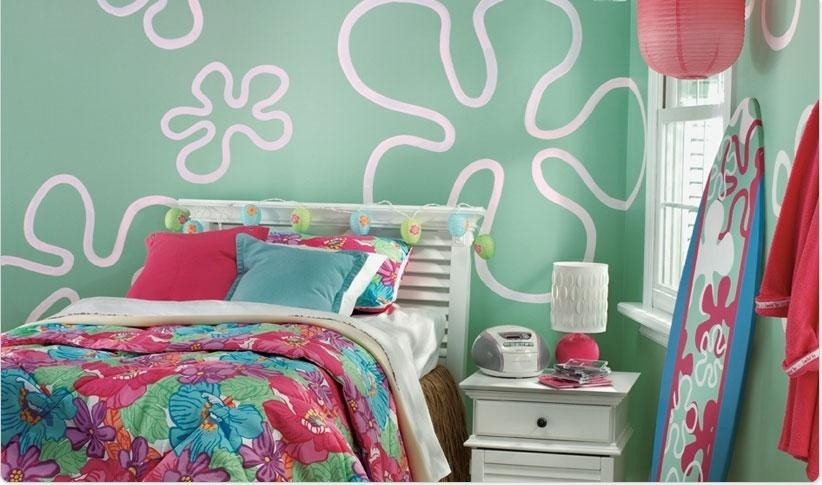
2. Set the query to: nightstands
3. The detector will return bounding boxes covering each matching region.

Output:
[456,367,642,481]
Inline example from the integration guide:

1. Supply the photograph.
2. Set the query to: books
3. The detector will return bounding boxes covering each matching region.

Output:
[539,358,613,389]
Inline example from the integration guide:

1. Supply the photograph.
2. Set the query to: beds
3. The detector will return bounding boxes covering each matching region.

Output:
[0,197,485,482]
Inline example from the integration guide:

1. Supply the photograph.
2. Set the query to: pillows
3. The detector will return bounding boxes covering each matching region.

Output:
[125,225,270,301]
[266,227,415,315]
[225,232,370,316]
[266,239,388,317]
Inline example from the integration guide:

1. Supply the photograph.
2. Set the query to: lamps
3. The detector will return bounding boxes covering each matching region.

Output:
[550,261,610,366]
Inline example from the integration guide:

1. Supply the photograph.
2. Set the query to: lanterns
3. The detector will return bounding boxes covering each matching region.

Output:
[635,0,745,80]
